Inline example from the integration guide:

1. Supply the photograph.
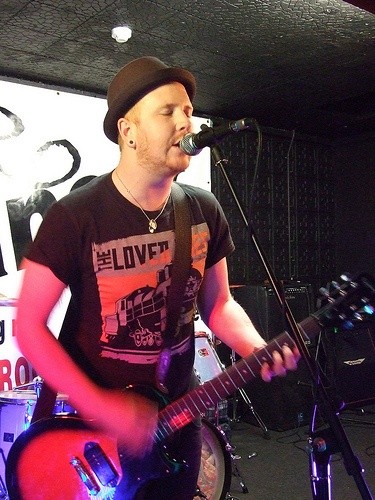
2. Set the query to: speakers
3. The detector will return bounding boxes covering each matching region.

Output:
[227,281,375,432]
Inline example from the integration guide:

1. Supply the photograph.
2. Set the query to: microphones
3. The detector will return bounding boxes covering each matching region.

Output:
[181,118,255,156]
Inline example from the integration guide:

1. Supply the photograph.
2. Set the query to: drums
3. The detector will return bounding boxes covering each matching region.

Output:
[0,389,78,500]
[186,331,227,413]
[194,416,234,500]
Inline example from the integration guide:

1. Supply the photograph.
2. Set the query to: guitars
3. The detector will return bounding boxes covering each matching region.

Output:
[5,274,375,500]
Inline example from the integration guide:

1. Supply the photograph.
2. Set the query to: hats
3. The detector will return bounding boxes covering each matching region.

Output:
[103,56,196,145]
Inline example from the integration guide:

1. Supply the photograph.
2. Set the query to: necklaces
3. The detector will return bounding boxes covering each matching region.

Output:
[115,167,171,233]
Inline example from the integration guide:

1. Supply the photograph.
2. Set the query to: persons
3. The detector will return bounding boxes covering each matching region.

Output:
[15,56,301,500]
